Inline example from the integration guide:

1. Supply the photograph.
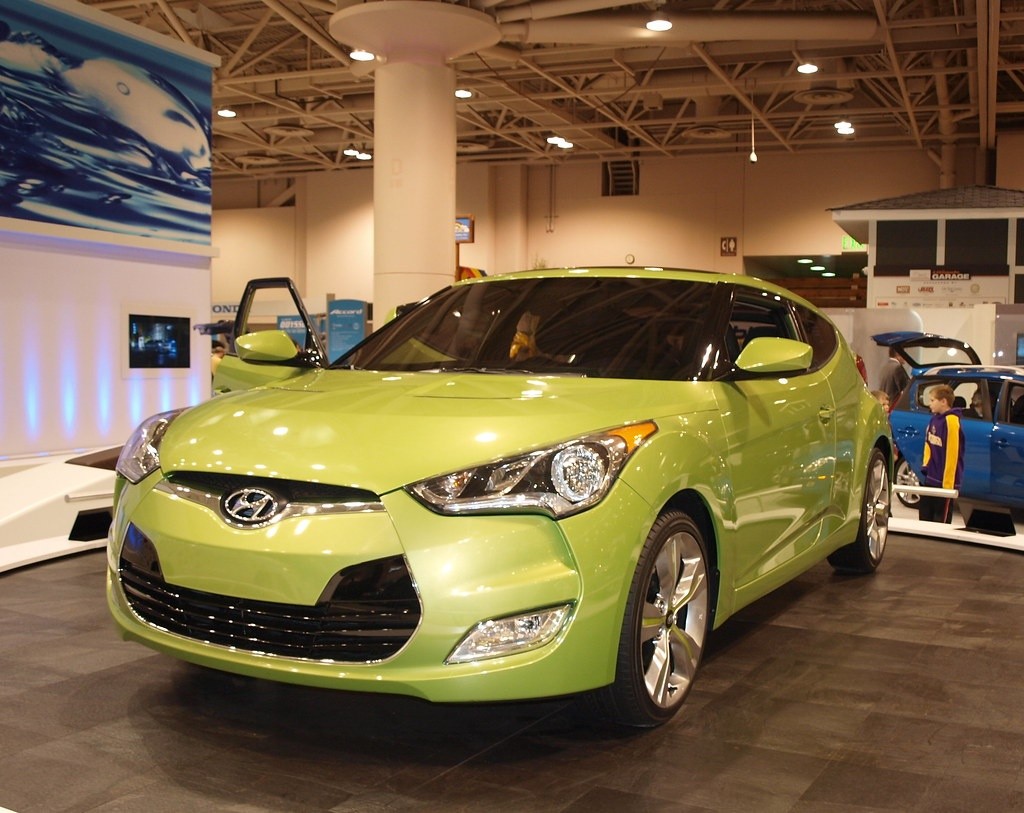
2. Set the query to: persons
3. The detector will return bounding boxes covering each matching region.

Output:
[919,385,965,524]
[879,341,910,409]
[869,390,898,464]
[968,388,995,420]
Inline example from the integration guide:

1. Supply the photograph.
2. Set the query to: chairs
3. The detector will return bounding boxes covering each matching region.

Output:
[1010,395,1023,425]
[952,396,966,409]
[682,311,740,364]
[510,306,626,378]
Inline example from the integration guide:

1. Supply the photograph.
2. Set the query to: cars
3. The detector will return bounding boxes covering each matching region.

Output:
[870,330,1023,513]
[104,276,896,728]
